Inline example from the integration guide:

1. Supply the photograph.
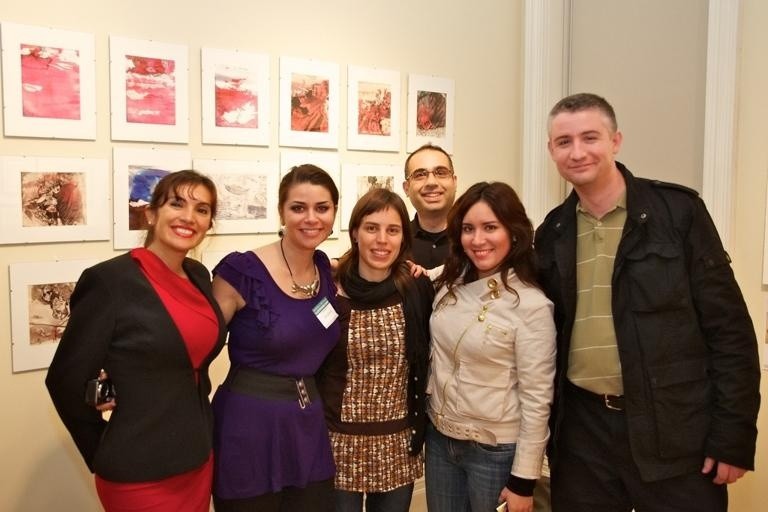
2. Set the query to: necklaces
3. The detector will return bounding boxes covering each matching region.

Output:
[279,237,321,298]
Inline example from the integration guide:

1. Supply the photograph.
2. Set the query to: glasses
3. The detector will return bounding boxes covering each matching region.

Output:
[407,168,454,180]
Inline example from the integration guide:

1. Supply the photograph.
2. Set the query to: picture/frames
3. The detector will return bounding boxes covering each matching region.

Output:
[341,165,420,231]
[1,21,96,141]
[193,159,280,235]
[408,71,456,157]
[199,48,271,147]
[346,64,401,153]
[278,55,339,151]
[8,262,99,376]
[113,147,192,253]
[0,157,111,246]
[108,36,191,144]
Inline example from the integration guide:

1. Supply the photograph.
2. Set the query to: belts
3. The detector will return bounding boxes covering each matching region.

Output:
[565,379,626,413]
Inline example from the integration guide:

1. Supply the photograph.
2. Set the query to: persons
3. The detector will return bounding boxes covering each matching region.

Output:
[94,163,342,511]
[330,143,457,279]
[534,92,761,512]
[45,170,226,512]
[315,187,433,511]
[426,181,557,512]
